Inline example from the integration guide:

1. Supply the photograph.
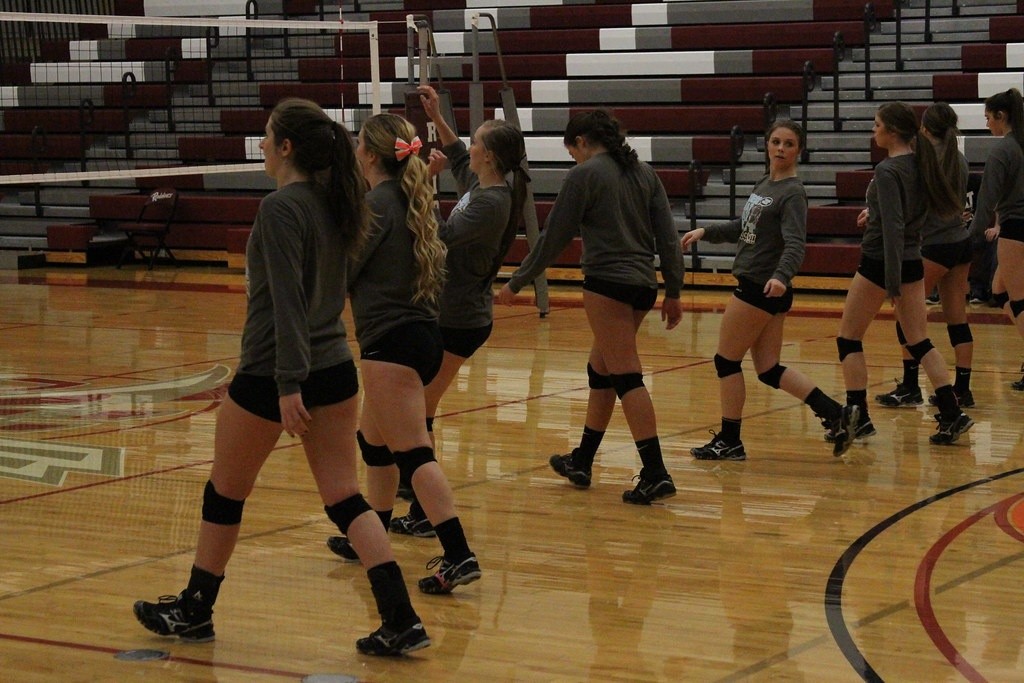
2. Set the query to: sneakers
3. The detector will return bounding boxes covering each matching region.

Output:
[690,428,748,461]
[417,552,483,594]
[133,590,218,641]
[874,378,924,406]
[824,412,876,443]
[969,291,985,304]
[929,409,973,445]
[356,621,434,656]
[550,453,591,488]
[389,515,436,538]
[829,404,859,456]
[327,536,362,562]
[931,389,976,408]
[925,294,940,303]
[620,467,676,504]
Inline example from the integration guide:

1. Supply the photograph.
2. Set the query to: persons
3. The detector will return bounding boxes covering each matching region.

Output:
[876,88,1024,407]
[133,96,430,656]
[681,119,861,462]
[327,114,483,594]
[499,108,686,503]
[822,102,975,444]
[386,85,532,536]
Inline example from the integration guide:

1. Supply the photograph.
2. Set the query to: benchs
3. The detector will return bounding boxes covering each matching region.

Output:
[0,0,1024,274]
